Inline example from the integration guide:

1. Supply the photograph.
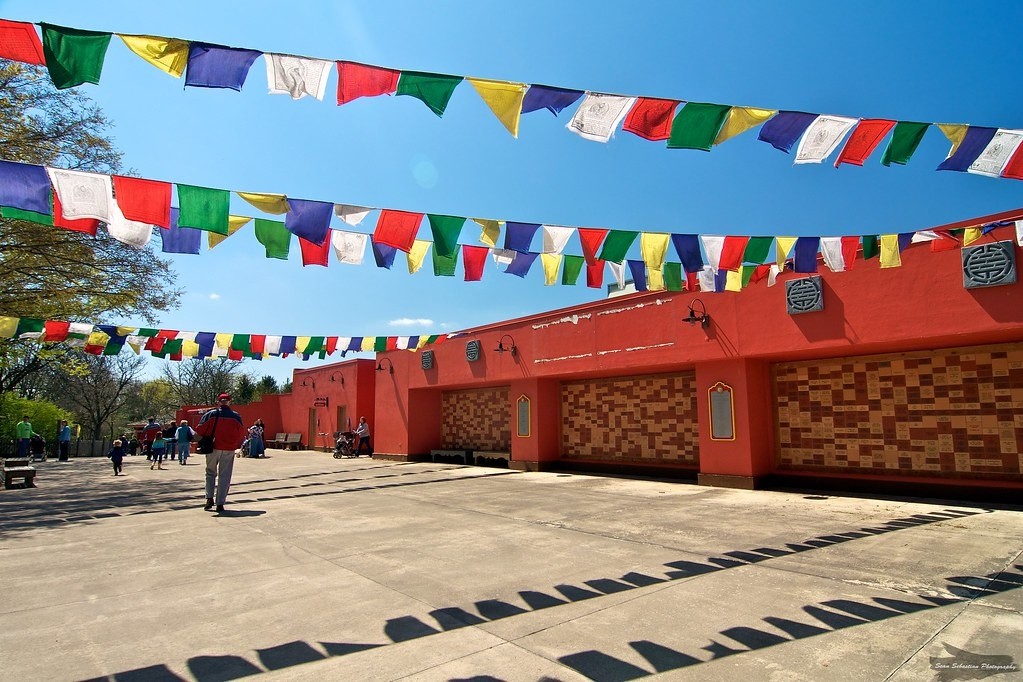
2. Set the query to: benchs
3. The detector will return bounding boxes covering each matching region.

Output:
[5,457,28,466]
[266,433,287,449]
[472,451,511,466]
[4,465,36,486]
[430,450,468,464]
[279,434,302,450]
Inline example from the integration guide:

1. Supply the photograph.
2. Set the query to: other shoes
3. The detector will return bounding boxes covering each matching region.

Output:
[158,467,162,470]
[205,499,214,509]
[171,458,174,460]
[179,461,182,464]
[183,463,186,465]
[216,505,224,513]
[151,465,153,470]
[163,456,167,458]
[333,449,339,453]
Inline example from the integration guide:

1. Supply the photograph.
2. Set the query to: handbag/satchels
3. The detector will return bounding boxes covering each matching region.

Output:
[186,427,194,442]
[196,437,213,454]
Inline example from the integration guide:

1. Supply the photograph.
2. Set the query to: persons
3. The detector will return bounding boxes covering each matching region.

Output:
[107,440,127,476]
[195,394,243,512]
[58,419,71,461]
[15,415,40,458]
[247,418,266,457]
[140,417,198,471]
[354,416,372,458]
[120,435,140,456]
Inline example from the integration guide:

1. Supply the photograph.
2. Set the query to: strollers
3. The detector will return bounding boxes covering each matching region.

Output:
[29,434,47,462]
[236,433,264,458]
[332,430,358,459]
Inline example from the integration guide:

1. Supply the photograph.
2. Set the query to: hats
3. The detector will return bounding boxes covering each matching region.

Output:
[218,393,231,403]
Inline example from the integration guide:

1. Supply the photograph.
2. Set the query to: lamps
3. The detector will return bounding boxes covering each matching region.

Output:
[375,357,393,375]
[682,299,709,329]
[329,371,344,384]
[493,335,517,357]
[300,376,315,390]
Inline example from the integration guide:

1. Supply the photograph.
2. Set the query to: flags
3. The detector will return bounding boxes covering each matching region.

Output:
[1,19,1023,180]
[0,162,1022,273]
[431,244,801,293]
[0,315,467,361]
